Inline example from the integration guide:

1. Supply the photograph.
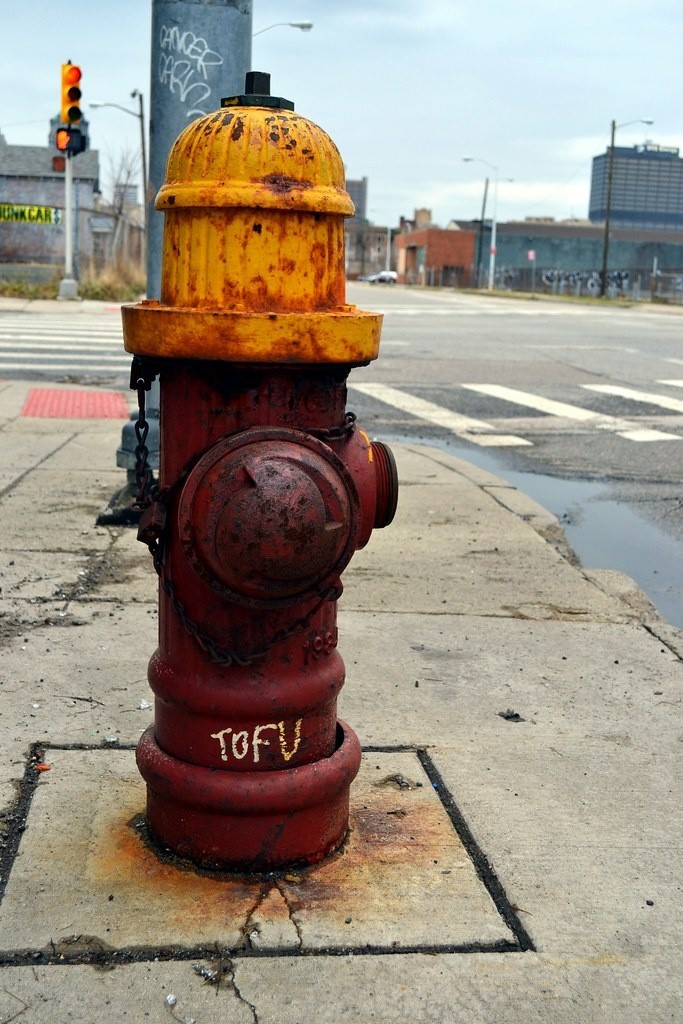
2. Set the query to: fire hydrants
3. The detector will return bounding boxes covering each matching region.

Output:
[95,69,402,882]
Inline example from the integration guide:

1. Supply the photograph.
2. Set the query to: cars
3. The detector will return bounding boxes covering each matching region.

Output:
[366,273,396,286]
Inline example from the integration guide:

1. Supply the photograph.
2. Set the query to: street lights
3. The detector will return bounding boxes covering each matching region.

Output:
[90,90,147,199]
[462,156,500,291]
[596,118,655,301]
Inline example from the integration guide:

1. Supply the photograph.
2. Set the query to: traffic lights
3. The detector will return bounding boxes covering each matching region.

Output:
[55,128,86,157]
[60,63,83,126]
